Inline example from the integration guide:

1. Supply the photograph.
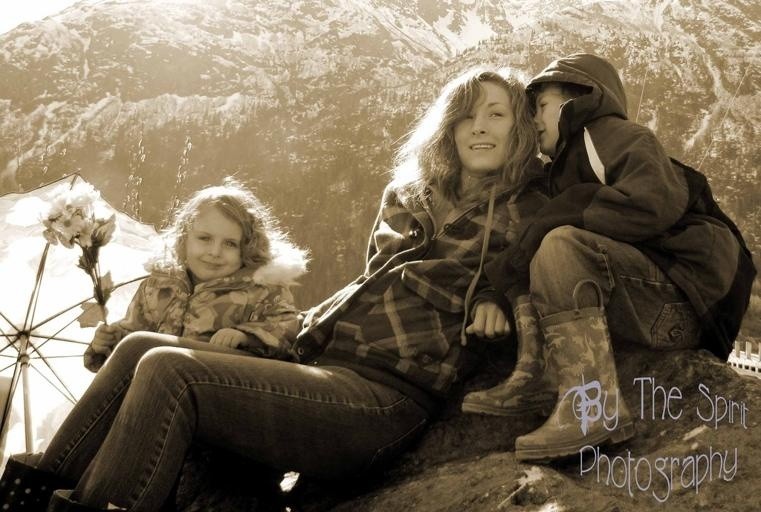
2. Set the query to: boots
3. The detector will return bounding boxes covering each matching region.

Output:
[461,293,638,461]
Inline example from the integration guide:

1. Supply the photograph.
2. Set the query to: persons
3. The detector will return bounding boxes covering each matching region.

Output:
[80,176,312,378]
[460,50,759,466]
[0,69,548,511]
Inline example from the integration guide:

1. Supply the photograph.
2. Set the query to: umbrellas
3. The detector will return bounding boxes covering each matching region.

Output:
[0,168,176,483]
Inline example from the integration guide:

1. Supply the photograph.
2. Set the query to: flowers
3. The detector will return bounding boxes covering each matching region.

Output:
[35,184,132,343]
